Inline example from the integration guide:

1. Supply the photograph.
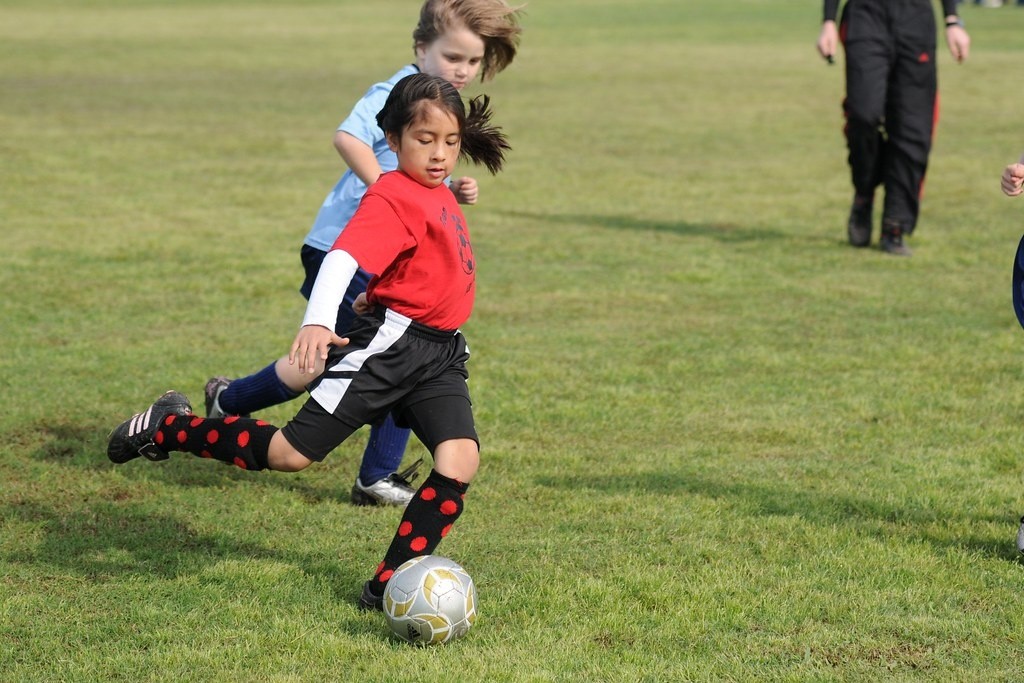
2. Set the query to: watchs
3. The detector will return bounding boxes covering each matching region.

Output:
[946,18,967,28]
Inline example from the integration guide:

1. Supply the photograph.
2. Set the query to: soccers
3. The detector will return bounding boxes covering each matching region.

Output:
[381,554,481,645]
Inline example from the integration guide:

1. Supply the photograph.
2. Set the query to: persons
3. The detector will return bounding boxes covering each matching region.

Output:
[204,0,523,508]
[815,0,970,255]
[1001,154,1024,328]
[106,72,514,611]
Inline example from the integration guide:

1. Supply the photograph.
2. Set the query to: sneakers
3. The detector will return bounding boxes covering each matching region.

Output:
[880,234,913,256]
[204,377,251,420]
[351,453,426,507]
[849,200,872,247]
[358,580,383,612]
[105,390,192,464]
